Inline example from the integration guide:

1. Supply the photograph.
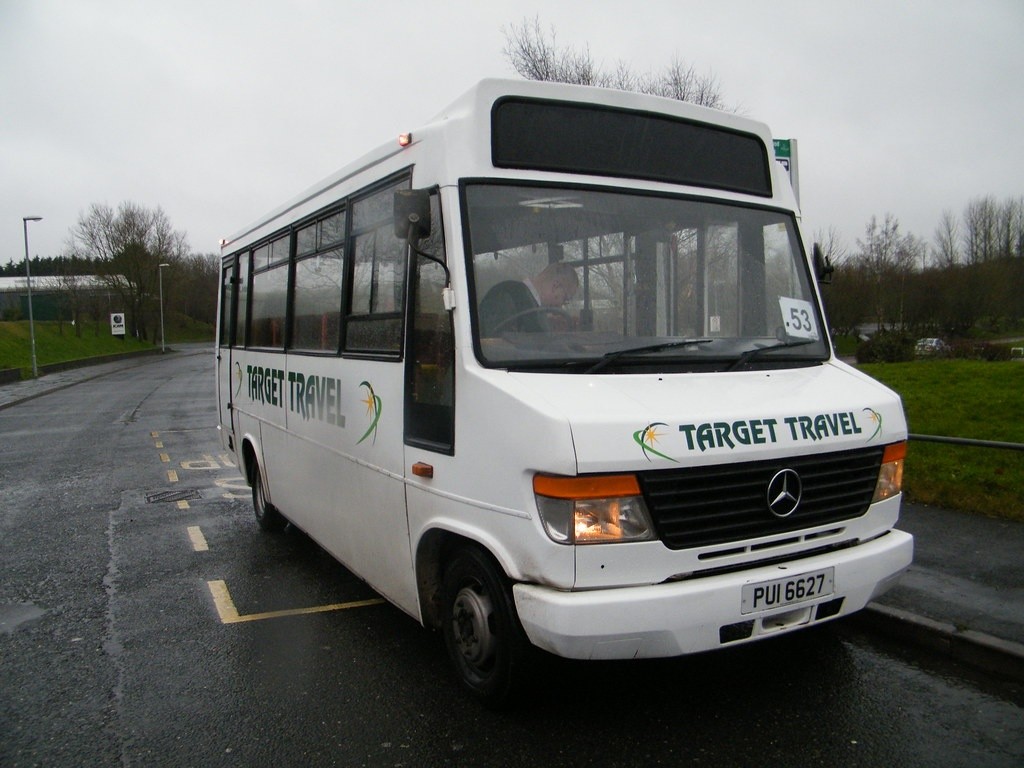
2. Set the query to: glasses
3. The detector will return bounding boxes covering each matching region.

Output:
[555,279,572,305]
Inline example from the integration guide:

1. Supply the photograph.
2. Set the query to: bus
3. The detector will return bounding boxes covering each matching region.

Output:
[214,77,915,711]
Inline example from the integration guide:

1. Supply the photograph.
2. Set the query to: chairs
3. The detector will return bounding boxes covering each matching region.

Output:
[254,308,585,421]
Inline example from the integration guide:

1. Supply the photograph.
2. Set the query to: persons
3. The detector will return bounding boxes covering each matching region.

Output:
[478,262,579,339]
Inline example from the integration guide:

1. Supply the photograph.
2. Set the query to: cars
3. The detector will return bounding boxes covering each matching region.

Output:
[914,339,950,357]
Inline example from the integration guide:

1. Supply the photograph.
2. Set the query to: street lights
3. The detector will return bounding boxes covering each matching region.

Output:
[159,263,170,354]
[23,216,43,378]
